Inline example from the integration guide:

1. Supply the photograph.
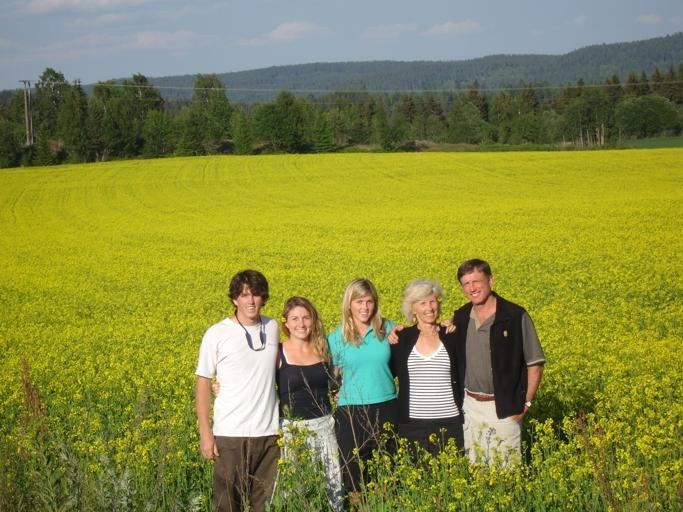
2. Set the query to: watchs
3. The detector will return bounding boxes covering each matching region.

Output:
[524,400,532,408]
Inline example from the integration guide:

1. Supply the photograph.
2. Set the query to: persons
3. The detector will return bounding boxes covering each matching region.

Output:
[193,267,279,512]
[211,294,344,512]
[387,257,547,476]
[334,279,466,473]
[328,278,458,511]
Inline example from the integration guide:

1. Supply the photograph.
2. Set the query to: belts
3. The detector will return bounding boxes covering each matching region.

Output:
[467,390,495,401]
